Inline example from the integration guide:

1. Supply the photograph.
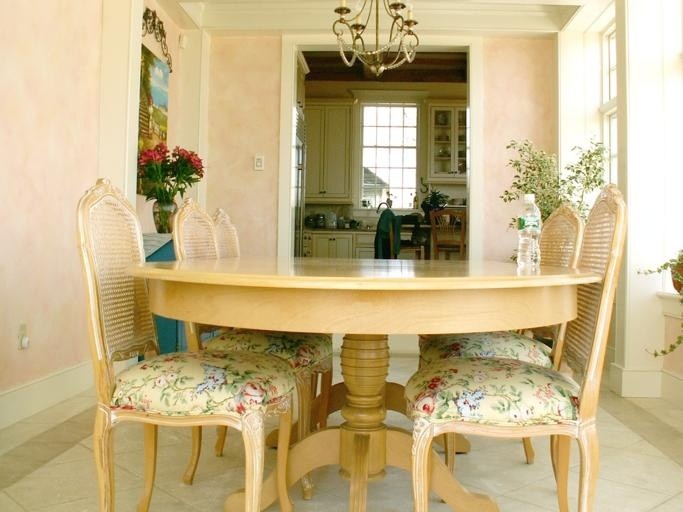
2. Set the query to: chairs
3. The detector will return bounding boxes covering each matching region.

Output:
[213,207,337,436]
[390,215,424,260]
[403,185,628,512]
[175,197,332,504]
[430,209,466,261]
[418,217,544,340]
[417,204,580,464]
[79,179,294,512]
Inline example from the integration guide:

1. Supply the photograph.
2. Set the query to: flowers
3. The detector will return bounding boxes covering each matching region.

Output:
[138,145,205,201]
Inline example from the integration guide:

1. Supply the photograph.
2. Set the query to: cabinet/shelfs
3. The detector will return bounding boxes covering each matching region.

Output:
[355,231,469,264]
[428,98,472,184]
[312,229,352,259]
[304,98,357,205]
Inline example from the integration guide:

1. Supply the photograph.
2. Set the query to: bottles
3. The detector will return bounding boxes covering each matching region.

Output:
[516,195,541,277]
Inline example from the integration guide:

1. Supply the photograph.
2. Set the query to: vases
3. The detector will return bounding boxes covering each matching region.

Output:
[150,199,179,236]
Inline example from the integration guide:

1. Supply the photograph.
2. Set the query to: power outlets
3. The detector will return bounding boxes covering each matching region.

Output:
[18,323,31,349]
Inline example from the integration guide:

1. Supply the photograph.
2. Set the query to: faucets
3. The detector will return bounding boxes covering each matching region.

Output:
[376,202,390,214]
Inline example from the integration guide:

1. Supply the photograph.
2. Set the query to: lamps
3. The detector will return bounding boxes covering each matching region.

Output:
[330,0,419,76]
